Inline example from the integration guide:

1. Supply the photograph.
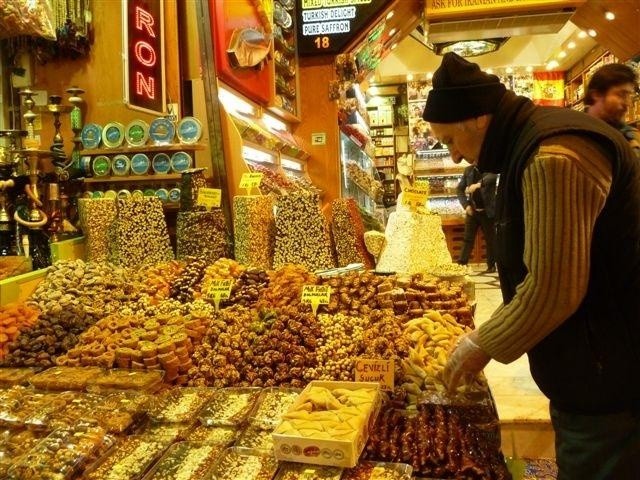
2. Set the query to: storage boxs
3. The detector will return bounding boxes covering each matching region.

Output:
[272,379,382,468]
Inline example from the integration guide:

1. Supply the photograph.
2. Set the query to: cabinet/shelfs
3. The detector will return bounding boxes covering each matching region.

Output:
[266,0,301,123]
[80,144,204,210]
[565,50,640,130]
[370,123,397,205]
[216,76,307,204]
[413,150,487,262]
[339,130,388,230]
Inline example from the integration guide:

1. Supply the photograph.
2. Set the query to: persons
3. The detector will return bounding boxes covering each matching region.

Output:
[574,63,640,157]
[455,161,498,274]
[421,51,639,478]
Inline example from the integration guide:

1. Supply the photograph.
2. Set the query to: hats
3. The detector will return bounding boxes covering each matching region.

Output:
[422,50,508,125]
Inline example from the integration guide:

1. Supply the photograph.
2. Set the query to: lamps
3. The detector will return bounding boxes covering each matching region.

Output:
[434,36,511,58]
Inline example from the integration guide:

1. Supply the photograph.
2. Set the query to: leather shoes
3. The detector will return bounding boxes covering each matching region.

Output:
[481,267,495,273]
